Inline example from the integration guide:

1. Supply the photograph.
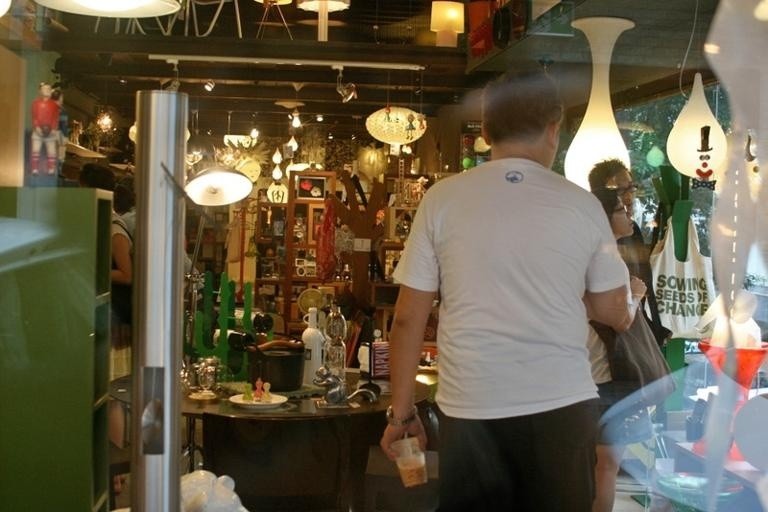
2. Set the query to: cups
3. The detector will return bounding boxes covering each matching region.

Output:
[391,438,429,489]
[195,358,219,390]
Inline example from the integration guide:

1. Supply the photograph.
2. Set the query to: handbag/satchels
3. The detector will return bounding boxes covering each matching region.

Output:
[111,250,133,324]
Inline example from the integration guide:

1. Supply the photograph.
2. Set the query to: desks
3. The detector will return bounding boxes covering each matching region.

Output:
[112,370,433,509]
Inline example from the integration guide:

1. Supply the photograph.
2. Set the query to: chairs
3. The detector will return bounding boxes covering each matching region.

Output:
[201,404,351,510]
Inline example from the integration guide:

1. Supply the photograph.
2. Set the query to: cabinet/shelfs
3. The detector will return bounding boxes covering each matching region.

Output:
[2,186,116,512]
[253,168,455,349]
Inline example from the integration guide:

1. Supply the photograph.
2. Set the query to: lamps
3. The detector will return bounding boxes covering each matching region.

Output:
[563,14,728,195]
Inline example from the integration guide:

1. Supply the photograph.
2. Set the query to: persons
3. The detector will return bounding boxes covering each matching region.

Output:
[379,63,648,512]
[588,159,674,347]
[587,189,655,512]
[114,176,200,289]
[31,82,68,175]
[79,164,134,495]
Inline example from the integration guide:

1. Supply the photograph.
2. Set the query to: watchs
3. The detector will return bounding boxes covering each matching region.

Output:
[386,404,418,427]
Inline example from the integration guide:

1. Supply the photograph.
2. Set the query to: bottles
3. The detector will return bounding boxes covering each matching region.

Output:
[302,306,346,382]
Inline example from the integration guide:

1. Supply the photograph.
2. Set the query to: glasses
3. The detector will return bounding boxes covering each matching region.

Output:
[612,205,628,212]
[616,185,638,192]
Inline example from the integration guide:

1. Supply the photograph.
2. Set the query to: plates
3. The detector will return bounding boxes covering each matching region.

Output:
[230,393,288,410]
